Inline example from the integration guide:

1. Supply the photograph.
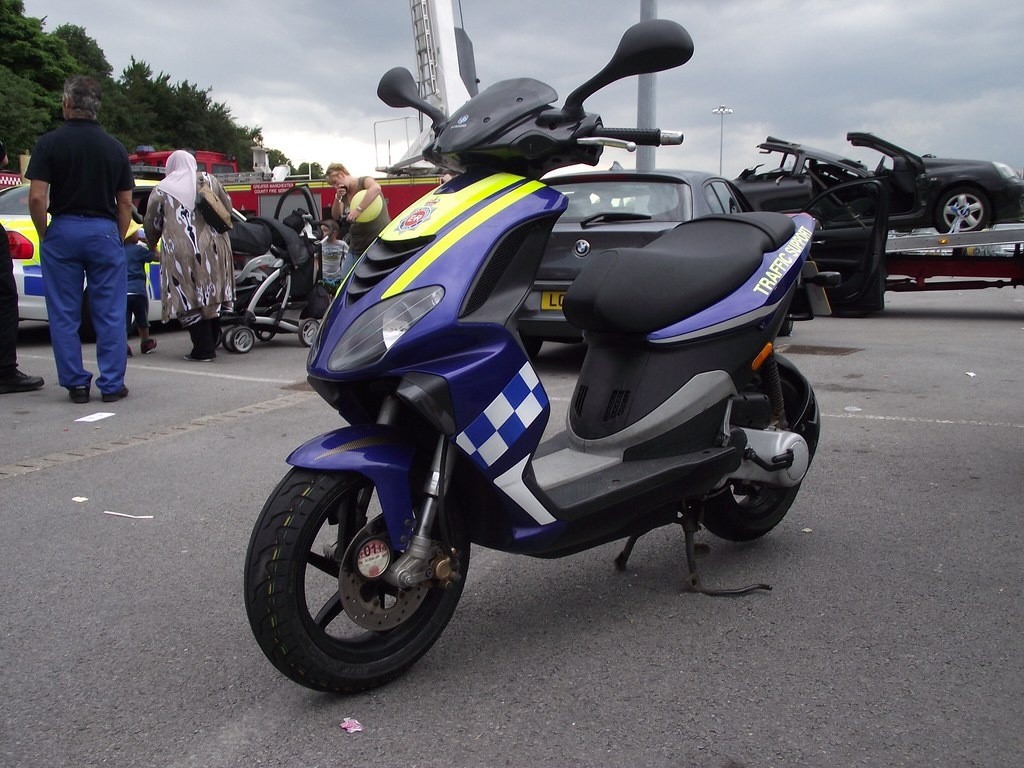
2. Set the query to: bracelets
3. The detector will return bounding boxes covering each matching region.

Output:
[357,207,363,212]
[335,193,342,202]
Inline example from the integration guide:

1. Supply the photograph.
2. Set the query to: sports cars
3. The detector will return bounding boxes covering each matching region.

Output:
[731,132,1024,234]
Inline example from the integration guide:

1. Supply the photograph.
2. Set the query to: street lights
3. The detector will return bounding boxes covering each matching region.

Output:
[711,105,734,176]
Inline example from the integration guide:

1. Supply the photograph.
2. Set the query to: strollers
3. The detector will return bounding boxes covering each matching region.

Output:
[221,185,320,354]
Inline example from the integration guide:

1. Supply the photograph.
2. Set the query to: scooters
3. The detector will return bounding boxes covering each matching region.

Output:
[246,21,822,698]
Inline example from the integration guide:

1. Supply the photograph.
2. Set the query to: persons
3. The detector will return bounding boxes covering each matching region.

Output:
[143,150,233,362]
[124,220,160,358]
[314,220,349,301]
[325,163,391,280]
[0,142,44,394]
[24,75,136,404]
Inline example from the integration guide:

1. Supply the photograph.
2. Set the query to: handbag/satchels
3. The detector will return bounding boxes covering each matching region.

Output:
[194,176,232,233]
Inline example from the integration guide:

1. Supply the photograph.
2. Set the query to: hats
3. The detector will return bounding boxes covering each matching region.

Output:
[124,218,147,240]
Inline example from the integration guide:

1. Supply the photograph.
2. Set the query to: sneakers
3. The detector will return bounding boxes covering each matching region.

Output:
[102,384,129,402]
[140,338,158,354]
[69,388,92,403]
[126,345,133,358]
[0,369,45,394]
[184,353,215,362]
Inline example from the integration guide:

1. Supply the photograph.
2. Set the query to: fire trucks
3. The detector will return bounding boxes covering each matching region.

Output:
[130,146,457,224]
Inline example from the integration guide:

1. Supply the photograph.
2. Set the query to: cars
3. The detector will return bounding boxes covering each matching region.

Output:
[0,180,251,340]
[514,170,757,359]
